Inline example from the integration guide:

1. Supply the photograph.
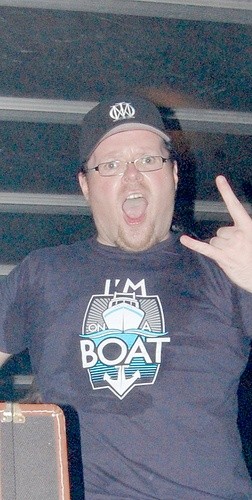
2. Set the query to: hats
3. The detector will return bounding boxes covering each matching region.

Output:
[79,95,172,171]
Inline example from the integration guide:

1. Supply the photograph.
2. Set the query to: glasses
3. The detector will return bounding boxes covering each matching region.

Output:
[82,155,173,178]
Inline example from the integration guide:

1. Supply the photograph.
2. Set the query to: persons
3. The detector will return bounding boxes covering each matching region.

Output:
[0,94,251,500]
